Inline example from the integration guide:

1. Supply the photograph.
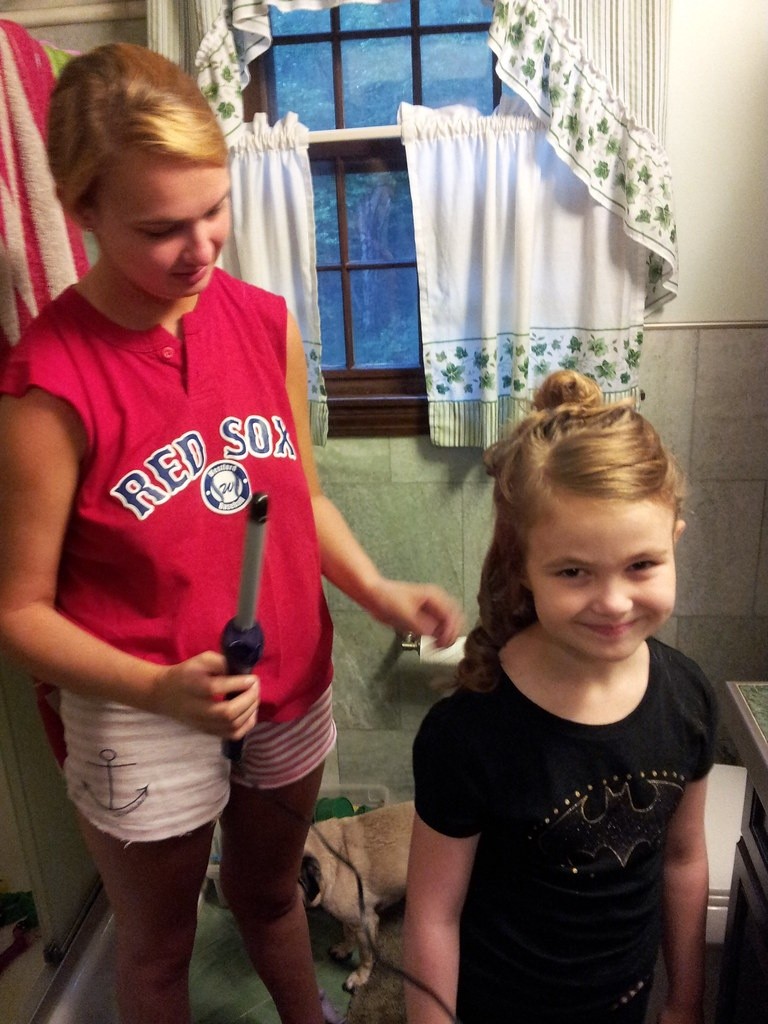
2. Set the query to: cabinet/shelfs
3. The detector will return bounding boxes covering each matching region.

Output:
[715,775,768,1024]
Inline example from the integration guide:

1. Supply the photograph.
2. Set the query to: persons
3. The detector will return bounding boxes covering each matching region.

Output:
[400,371,721,1023]
[1,41,466,1024]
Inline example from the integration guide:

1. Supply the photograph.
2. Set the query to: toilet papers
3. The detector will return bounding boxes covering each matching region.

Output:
[418,634,466,668]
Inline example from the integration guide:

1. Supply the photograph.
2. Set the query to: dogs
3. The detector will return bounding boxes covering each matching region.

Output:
[297,801,415,991]
